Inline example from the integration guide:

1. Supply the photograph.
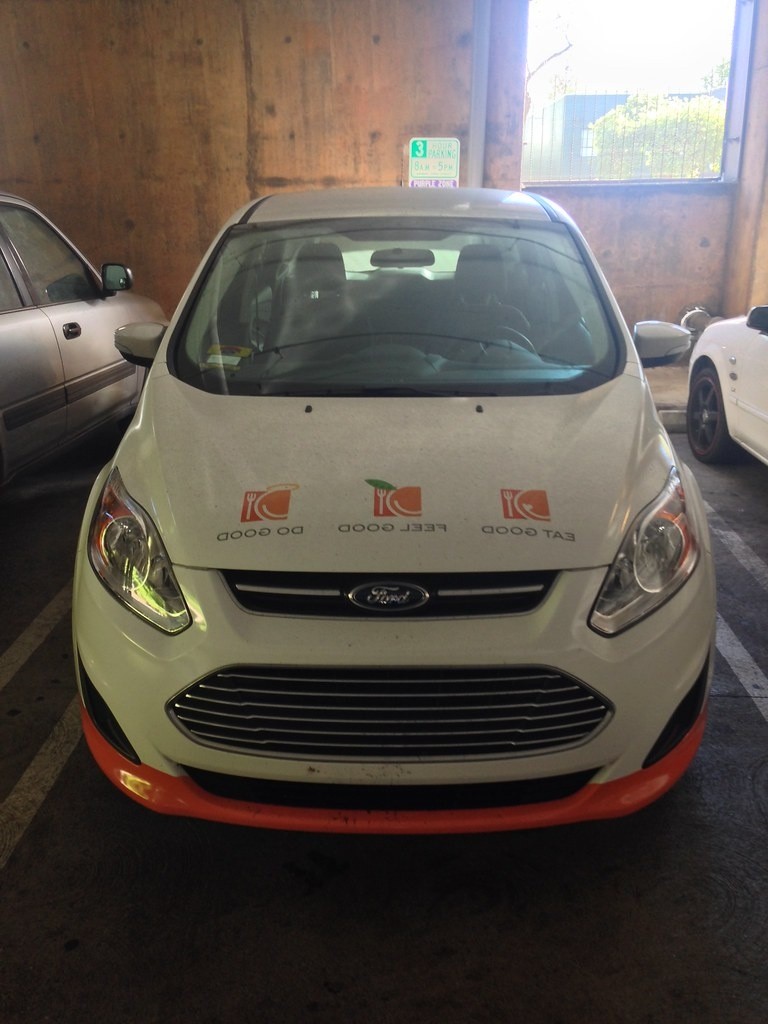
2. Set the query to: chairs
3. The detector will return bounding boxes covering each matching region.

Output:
[435,243,529,352]
[278,244,352,348]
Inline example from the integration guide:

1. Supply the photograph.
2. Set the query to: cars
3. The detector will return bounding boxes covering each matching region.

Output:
[1,192,172,488]
[685,305,768,468]
[69,183,720,838]
